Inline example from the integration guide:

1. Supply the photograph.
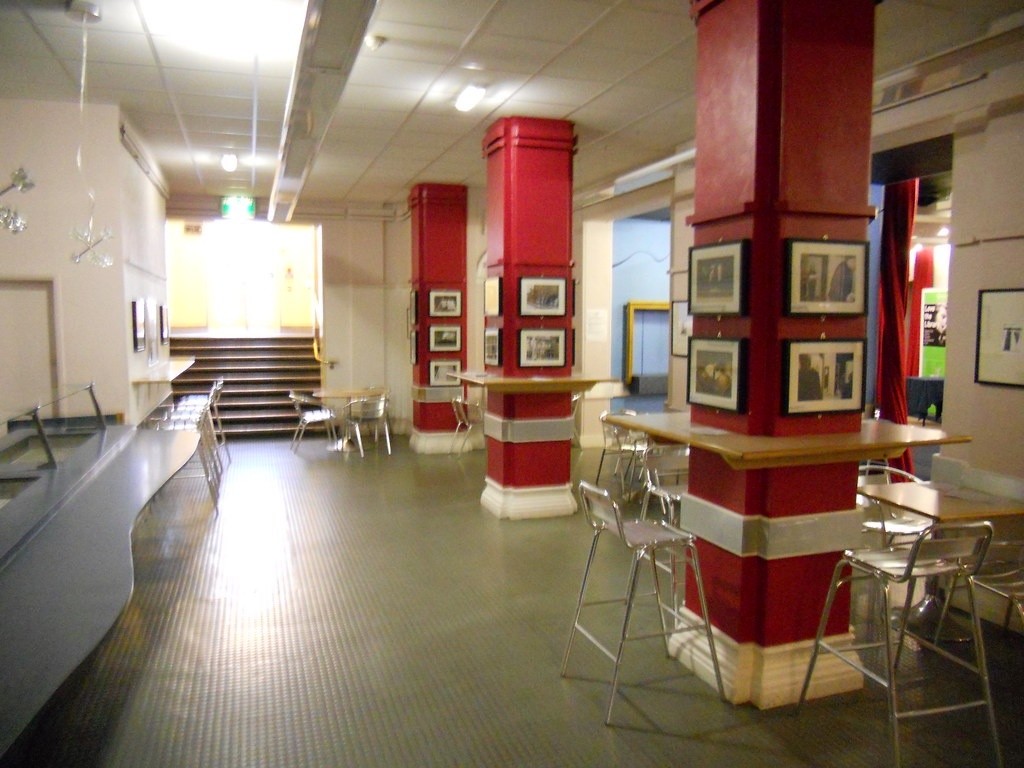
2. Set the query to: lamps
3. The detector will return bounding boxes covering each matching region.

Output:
[39,1,116,271]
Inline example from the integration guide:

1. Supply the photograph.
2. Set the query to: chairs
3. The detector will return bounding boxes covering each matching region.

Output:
[153,375,230,516]
[289,384,394,457]
[571,393,1024,768]
[450,398,484,456]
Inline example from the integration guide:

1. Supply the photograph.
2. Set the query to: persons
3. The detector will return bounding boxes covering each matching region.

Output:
[797,354,823,401]
[829,256,856,303]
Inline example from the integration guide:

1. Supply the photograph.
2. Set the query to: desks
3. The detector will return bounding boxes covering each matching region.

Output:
[852,482,1024,642]
[448,373,499,387]
[312,390,384,452]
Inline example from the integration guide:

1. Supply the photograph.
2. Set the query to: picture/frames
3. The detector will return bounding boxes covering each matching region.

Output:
[406,291,418,365]
[688,239,751,317]
[483,278,501,317]
[429,358,461,386]
[429,324,462,352]
[688,337,750,413]
[780,338,866,415]
[519,328,566,367]
[483,327,501,367]
[519,277,566,316]
[133,298,170,352]
[784,237,869,316]
[429,290,462,316]
[974,288,1024,388]
